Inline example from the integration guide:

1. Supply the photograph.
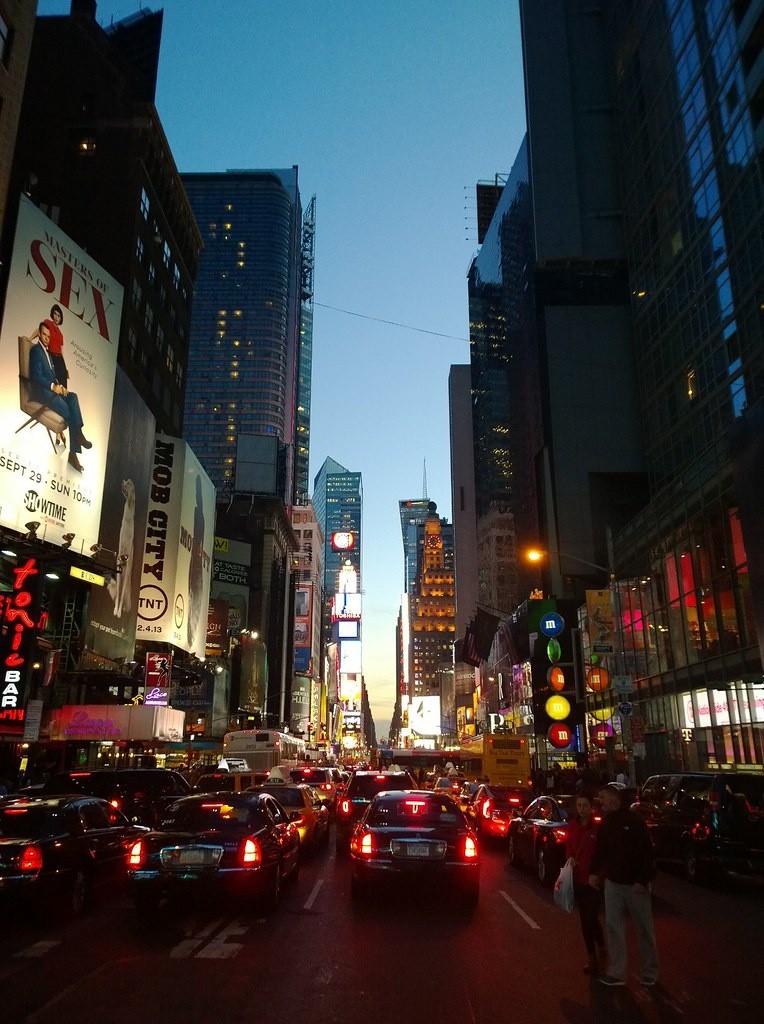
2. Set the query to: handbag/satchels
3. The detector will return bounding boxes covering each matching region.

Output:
[553,857,577,913]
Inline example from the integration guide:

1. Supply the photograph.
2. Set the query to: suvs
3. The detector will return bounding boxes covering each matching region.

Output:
[627,770,764,888]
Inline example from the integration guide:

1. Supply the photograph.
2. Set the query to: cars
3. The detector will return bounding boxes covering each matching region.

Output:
[506,795,608,886]
[420,769,537,838]
[350,790,480,902]
[0,794,150,921]
[335,770,420,831]
[0,758,352,857]
[127,791,302,916]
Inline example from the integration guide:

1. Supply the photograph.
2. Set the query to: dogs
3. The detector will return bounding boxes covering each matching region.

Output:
[107,478,135,618]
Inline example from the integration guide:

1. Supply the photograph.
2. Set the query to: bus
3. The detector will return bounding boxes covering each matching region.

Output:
[375,748,483,781]
[223,731,307,773]
[459,734,532,789]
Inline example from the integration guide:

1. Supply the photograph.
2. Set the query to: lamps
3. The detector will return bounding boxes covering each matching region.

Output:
[114,657,127,676]
[62,533,75,549]
[90,542,102,559]
[117,553,129,569]
[25,522,40,540]
[203,662,224,676]
[45,567,62,579]
[127,661,139,676]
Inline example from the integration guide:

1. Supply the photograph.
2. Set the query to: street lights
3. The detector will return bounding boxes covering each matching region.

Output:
[525,526,636,788]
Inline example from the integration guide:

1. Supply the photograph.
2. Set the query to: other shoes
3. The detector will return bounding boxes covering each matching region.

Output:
[640,976,655,985]
[599,975,627,985]
[596,952,606,973]
[582,953,600,972]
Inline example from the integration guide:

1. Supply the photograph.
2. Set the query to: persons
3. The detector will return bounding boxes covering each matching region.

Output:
[588,782,660,987]
[564,791,604,975]
[530,766,556,796]
[17,769,32,787]
[616,769,630,784]
[563,765,588,775]
[172,764,196,773]
[23,303,93,470]
[100,752,130,769]
[408,767,425,783]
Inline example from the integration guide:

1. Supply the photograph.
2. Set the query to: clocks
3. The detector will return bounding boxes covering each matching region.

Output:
[427,535,441,547]
